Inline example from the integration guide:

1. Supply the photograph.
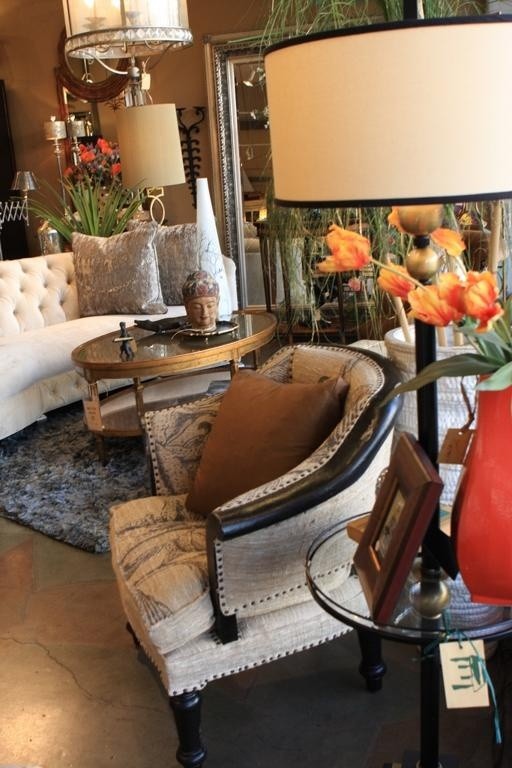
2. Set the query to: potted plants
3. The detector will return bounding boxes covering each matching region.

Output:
[348,514,371,544]
[352,432,445,628]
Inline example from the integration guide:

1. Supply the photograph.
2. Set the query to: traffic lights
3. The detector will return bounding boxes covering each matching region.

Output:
[185,365,348,515]
[72,224,167,318]
[130,220,200,306]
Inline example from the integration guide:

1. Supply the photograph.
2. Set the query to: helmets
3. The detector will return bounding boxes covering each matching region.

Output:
[175,321,240,336]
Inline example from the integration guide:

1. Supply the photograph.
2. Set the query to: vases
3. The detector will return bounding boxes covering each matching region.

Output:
[318,208,512,410]
[63,138,123,187]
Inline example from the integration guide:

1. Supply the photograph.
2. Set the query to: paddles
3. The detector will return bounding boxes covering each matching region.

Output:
[3,358,253,552]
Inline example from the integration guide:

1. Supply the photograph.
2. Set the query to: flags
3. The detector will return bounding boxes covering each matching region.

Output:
[197,177,233,315]
[89,189,111,225]
[451,372,511,606]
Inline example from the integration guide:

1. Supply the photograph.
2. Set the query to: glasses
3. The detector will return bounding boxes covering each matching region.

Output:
[67,112,87,163]
[62,1,192,211]
[44,116,68,217]
[115,103,187,226]
[264,12,512,765]
[12,170,36,198]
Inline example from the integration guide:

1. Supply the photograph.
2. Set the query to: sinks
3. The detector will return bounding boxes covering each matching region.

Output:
[109,342,406,768]
[2,250,237,442]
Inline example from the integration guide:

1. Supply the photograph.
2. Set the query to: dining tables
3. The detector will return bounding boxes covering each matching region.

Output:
[259,0,512,657]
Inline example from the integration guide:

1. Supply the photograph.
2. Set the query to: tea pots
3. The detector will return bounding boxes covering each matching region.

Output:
[55,61,103,213]
[205,14,391,367]
[57,27,133,103]
